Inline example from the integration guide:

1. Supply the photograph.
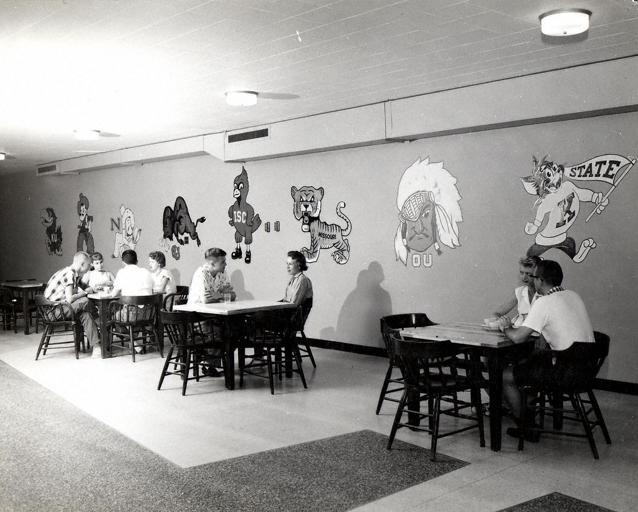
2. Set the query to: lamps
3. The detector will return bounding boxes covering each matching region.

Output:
[539,8,592,37]
[73,129,100,141]
[225,91,258,106]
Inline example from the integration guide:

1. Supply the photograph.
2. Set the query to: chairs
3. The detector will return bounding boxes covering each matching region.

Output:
[374,312,611,460]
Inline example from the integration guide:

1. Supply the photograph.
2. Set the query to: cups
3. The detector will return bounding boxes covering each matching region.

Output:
[489,322,501,328]
[107,281,113,290]
[224,294,232,303]
[484,318,496,325]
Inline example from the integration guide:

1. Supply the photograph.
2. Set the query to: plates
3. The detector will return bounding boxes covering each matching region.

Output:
[482,324,489,327]
[485,327,500,331]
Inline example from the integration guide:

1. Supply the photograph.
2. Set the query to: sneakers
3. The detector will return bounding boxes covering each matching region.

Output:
[506,423,542,444]
[128,342,154,354]
[92,347,111,358]
[202,367,221,377]
[93,317,112,328]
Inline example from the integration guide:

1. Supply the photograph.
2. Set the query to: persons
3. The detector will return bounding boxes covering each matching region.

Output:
[256,251,313,358]
[149,251,177,312]
[111,250,153,354]
[499,260,595,442]
[484,255,544,415]
[187,248,236,376]
[42,251,112,357]
[81,252,116,342]
